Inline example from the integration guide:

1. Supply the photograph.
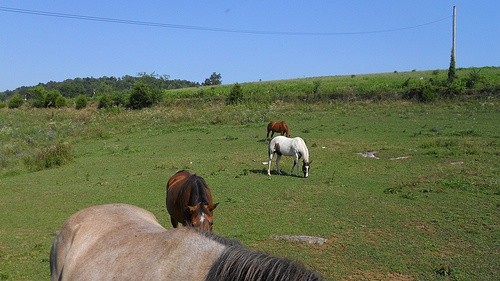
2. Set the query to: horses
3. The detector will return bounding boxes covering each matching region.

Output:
[267,136,313,178]
[50,202,327,281]
[266,121,291,138]
[166,169,221,234]
